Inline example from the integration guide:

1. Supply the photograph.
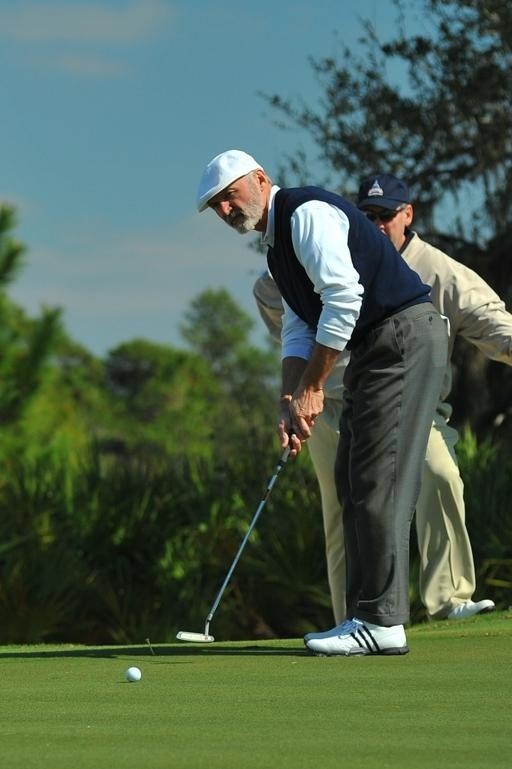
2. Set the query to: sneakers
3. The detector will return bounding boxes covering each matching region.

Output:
[303,616,409,657]
[427,599,496,622]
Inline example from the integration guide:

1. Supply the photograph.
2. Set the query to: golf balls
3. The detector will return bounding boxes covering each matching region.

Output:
[125,666,143,681]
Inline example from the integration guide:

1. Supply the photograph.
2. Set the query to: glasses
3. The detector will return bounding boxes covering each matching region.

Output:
[361,207,406,223]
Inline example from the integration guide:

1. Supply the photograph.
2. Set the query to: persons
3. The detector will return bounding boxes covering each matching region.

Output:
[194,148,451,658]
[250,172,511,626]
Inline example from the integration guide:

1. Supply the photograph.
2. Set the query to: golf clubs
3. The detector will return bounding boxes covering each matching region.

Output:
[174,430,292,642]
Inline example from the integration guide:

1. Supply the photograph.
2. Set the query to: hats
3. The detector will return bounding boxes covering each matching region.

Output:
[196,149,264,213]
[356,174,409,210]
